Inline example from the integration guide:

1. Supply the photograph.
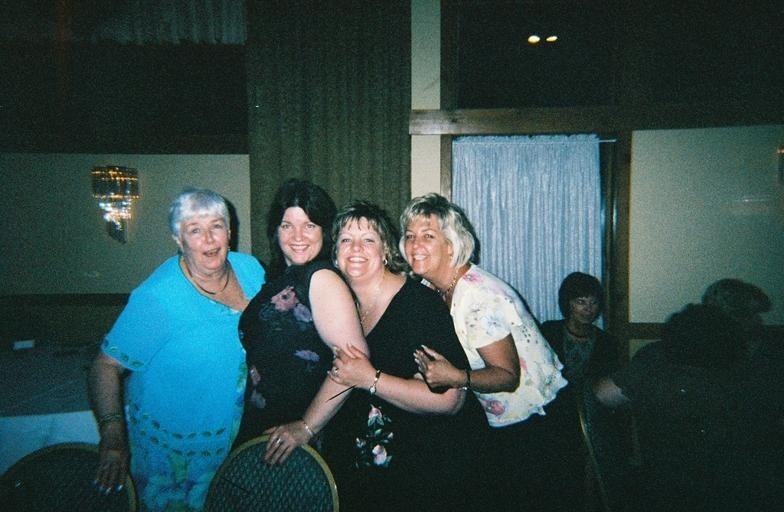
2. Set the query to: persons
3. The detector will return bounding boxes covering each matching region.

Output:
[535,273,784,512]
[89,188,268,512]
[229,177,371,465]
[398,193,578,512]
[324,201,492,511]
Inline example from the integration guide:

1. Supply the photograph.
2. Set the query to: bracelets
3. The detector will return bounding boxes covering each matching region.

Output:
[297,416,315,437]
[97,413,125,431]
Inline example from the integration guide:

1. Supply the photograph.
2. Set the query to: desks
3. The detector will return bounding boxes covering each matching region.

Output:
[0,339,125,464]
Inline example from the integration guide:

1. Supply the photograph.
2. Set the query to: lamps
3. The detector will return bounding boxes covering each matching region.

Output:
[88,164,140,245]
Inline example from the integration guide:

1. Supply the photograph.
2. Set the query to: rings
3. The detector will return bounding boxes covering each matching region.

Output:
[275,438,283,445]
[334,368,339,375]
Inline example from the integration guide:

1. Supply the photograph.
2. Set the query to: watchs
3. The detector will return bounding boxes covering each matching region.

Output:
[369,370,381,394]
[458,368,470,391]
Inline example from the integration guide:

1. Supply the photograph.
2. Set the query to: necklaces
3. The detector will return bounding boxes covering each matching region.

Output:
[183,258,230,295]
[438,270,457,296]
[357,272,386,324]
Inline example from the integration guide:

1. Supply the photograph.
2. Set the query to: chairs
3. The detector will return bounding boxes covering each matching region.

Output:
[204,432,342,511]
[572,367,686,511]
[0,440,142,512]
